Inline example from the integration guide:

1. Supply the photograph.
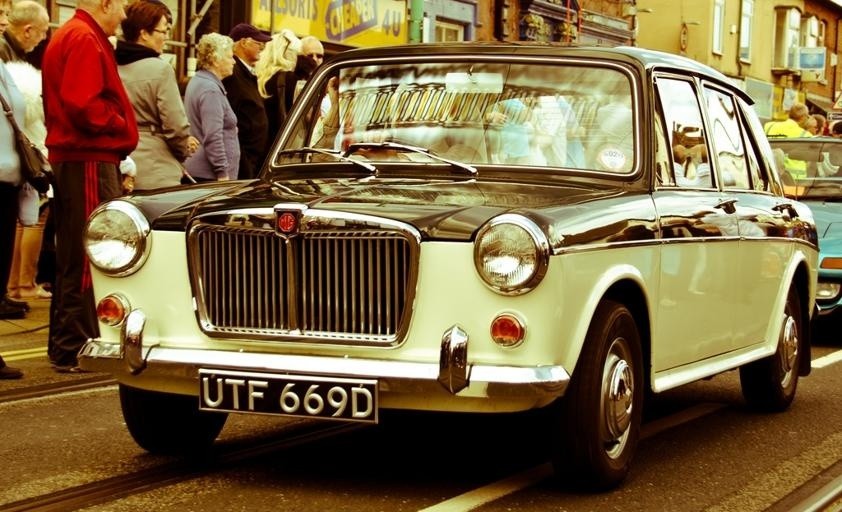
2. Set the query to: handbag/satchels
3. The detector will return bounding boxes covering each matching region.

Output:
[15,132,54,193]
[18,181,40,227]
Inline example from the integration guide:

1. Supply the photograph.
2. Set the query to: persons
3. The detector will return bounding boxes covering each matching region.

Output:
[301,36,324,67]
[306,76,339,163]
[424,75,634,174]
[0,0,49,65]
[253,30,317,149]
[115,0,200,191]
[0,0,26,380]
[181,32,241,184]
[764,103,842,196]
[222,24,273,180]
[39,0,139,375]
[6,193,52,299]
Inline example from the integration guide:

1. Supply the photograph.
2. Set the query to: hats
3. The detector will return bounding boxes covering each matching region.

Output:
[229,23,273,42]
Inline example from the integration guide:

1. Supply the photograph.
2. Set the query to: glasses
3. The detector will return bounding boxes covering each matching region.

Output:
[307,54,323,59]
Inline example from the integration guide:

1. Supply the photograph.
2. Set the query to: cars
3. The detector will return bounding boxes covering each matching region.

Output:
[768,134,842,317]
[76,39,819,489]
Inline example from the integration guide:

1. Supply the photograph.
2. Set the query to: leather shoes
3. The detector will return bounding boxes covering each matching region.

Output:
[0,284,51,319]
